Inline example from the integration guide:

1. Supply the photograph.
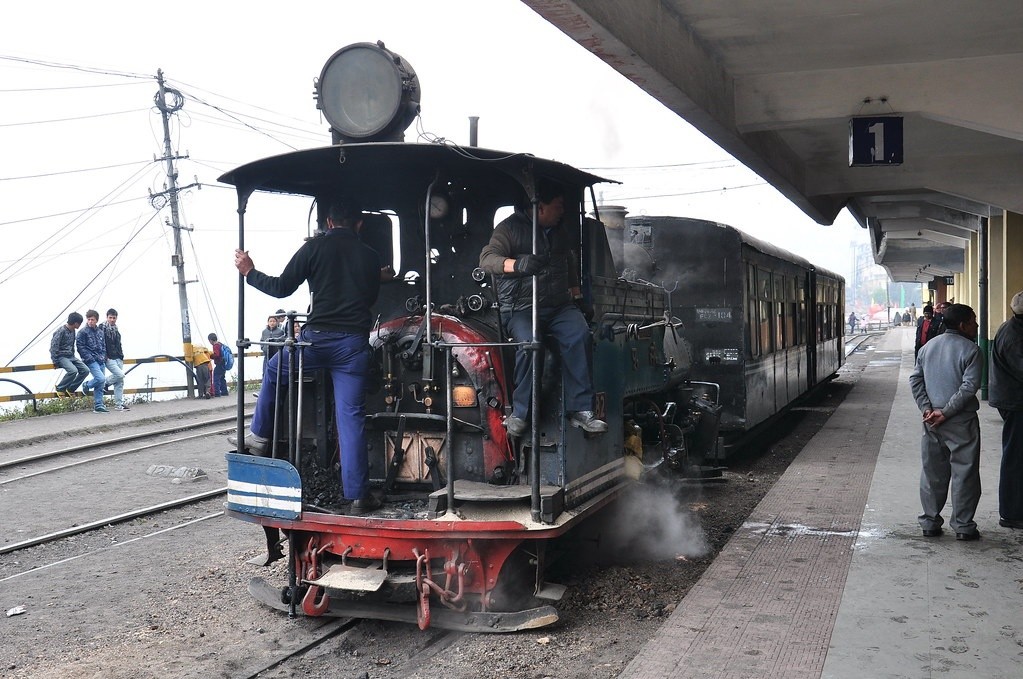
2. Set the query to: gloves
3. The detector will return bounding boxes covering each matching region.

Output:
[513,254,544,276]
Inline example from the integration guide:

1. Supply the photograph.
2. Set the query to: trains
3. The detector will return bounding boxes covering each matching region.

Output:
[214,39,846,633]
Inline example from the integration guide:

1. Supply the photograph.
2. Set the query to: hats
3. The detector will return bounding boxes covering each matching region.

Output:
[1011,292,1023,315]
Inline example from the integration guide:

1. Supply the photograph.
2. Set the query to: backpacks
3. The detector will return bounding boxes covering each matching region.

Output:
[220,344,234,371]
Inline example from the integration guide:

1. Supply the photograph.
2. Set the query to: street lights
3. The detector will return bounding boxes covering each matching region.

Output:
[850,240,858,314]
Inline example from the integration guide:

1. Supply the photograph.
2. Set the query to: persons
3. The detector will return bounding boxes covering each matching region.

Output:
[909,303,917,326]
[227,199,381,515]
[76,310,111,413]
[915,296,954,367]
[253,316,285,397]
[859,318,869,333]
[893,311,901,326]
[208,333,229,397]
[910,303,981,541]
[192,344,211,398]
[48,311,90,400]
[294,323,301,338]
[901,310,911,326]
[848,312,859,335]
[98,308,130,411]
[281,310,297,335]
[479,182,608,436]
[988,291,1023,528]
[274,309,286,328]
[204,351,215,397]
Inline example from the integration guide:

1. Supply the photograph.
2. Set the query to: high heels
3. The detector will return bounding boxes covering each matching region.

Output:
[227,433,268,456]
[351,488,385,515]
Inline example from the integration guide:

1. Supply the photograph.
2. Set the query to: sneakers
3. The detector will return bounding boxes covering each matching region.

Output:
[82,381,88,396]
[508,414,529,437]
[115,404,132,412]
[94,405,110,413]
[571,411,609,432]
[103,383,108,393]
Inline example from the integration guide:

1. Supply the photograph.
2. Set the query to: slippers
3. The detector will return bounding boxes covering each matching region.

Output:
[54,385,67,401]
[66,389,76,400]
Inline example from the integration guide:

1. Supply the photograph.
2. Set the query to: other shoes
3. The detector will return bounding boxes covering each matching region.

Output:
[206,392,211,398]
[195,395,204,399]
[956,529,980,540]
[923,529,943,537]
[999,517,1023,529]
